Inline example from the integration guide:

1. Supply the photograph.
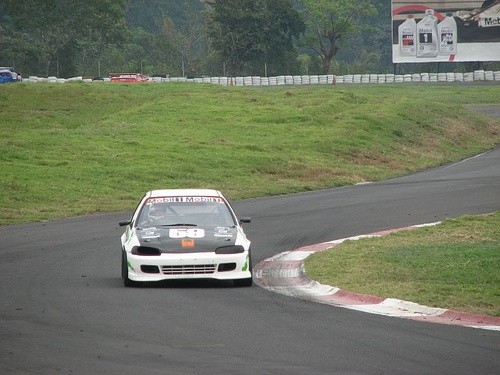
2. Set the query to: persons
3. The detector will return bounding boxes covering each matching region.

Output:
[141,205,168,226]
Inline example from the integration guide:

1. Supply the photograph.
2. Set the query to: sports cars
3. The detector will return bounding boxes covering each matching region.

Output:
[118,189,255,288]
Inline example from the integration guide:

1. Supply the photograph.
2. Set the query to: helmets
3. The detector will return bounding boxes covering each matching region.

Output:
[148,203,168,224]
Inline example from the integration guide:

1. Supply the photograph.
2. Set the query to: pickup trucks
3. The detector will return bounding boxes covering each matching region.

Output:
[110,73,157,83]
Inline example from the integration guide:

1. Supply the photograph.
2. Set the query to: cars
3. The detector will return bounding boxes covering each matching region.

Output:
[0,67,22,84]
